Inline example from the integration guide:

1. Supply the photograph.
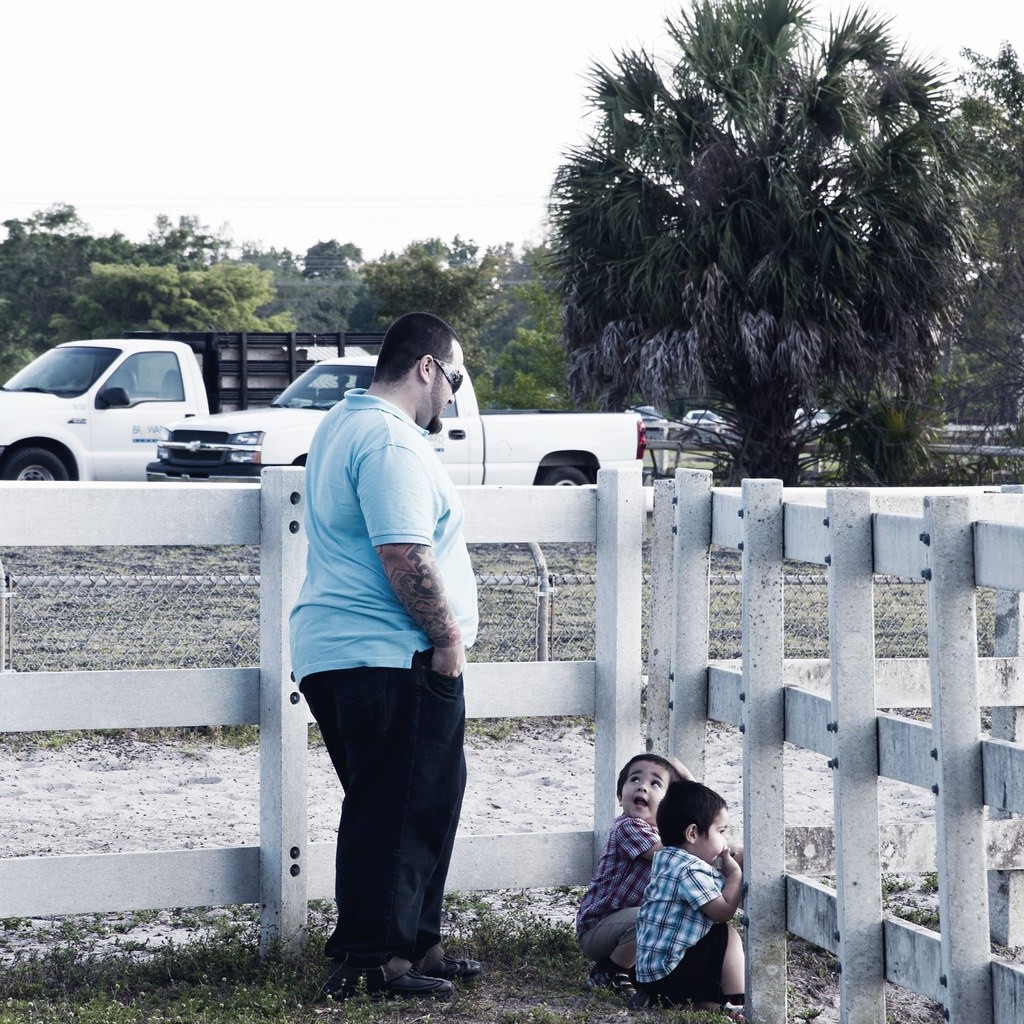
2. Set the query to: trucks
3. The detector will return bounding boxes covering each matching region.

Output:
[2,325,212,491]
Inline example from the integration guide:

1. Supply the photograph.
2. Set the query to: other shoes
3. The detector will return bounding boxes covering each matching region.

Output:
[721,1002,746,1024]
[680,999,722,1014]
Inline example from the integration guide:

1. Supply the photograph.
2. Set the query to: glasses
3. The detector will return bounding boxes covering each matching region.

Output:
[416,355,464,394]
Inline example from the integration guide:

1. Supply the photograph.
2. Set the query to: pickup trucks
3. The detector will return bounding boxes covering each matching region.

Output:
[158,352,650,509]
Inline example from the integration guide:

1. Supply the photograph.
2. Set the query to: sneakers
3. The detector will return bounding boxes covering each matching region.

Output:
[603,969,636,997]
[585,972,610,993]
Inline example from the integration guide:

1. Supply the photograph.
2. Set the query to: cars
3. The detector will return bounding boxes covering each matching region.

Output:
[626,398,849,445]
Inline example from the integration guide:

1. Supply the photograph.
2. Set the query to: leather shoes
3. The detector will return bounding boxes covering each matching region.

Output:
[422,952,482,984]
[323,965,453,1004]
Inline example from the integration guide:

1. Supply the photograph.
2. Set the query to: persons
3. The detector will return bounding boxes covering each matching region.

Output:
[576,754,696,998]
[633,781,745,1008]
[287,315,478,1001]
